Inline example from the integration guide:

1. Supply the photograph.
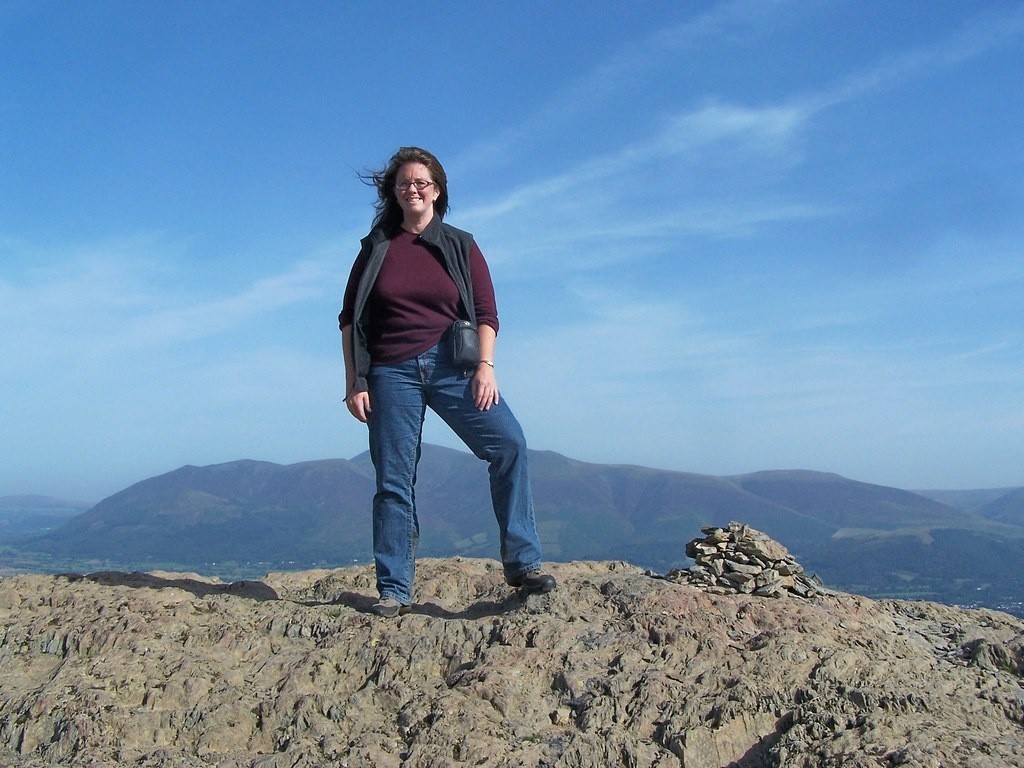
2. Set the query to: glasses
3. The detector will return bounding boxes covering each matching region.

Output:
[394,180,437,190]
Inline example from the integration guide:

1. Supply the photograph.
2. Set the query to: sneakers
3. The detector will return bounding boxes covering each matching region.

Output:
[506,567,556,590]
[371,596,412,618]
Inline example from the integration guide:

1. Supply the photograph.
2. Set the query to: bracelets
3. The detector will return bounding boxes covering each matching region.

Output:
[479,360,494,368]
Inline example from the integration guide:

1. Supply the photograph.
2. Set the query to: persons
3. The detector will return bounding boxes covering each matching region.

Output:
[338,147,557,619]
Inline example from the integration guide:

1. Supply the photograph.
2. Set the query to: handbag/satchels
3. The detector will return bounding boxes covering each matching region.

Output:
[452,321,482,366]
